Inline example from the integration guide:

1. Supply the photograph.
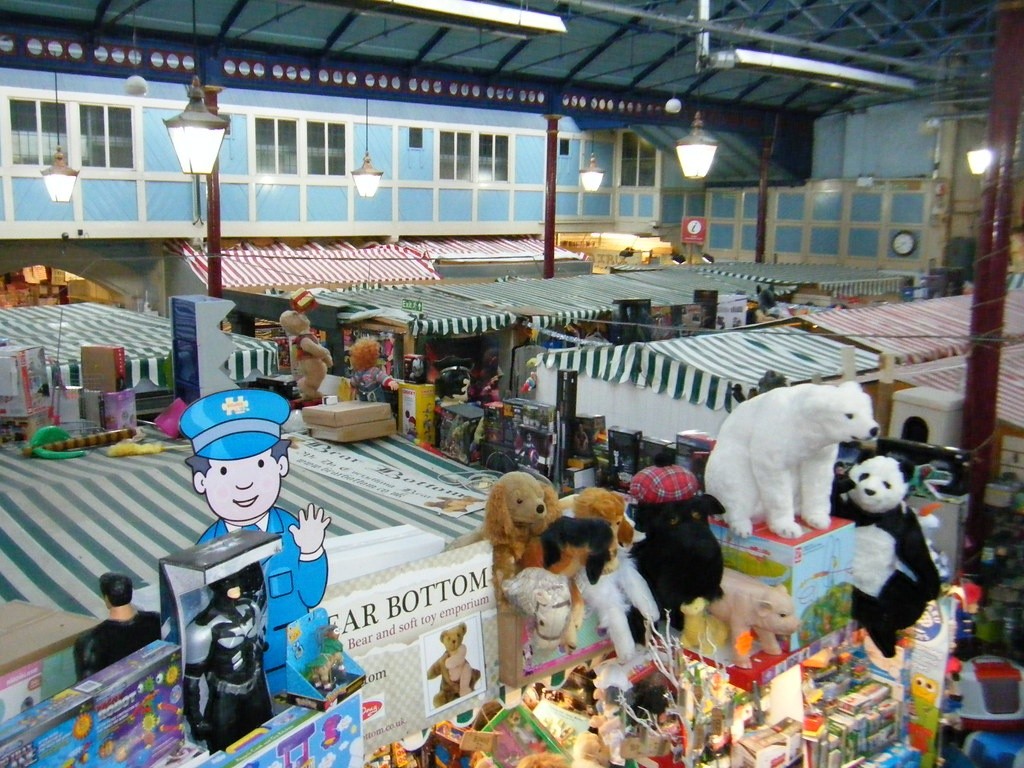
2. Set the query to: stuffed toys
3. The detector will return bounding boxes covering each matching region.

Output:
[280,310,333,401]
[437,472,660,664]
[830,449,941,659]
[349,338,400,403]
[710,566,802,669]
[625,454,725,645]
[427,623,482,708]
[502,666,688,768]
[704,379,881,540]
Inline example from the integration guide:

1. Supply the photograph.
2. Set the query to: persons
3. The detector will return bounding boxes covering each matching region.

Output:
[957,583,984,659]
[73,573,160,683]
[183,573,274,757]
[575,424,587,448]
[432,356,474,406]
[939,661,963,730]
[515,433,536,468]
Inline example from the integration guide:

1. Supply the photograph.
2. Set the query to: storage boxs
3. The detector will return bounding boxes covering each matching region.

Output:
[0,287,922,767]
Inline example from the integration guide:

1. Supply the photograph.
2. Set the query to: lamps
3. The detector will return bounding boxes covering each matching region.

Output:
[620,247,635,257]
[665,26,681,113]
[703,254,713,264]
[126,7,148,96]
[349,81,384,196]
[672,255,686,265]
[161,0,232,174]
[578,128,605,192]
[41,50,80,202]
[675,25,720,179]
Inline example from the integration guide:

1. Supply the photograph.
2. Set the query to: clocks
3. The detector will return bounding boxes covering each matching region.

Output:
[892,230,917,256]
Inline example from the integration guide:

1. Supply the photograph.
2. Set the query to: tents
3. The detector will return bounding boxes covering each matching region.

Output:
[0,416,499,621]
[163,235,902,414]
[536,288,1024,505]
[0,301,279,410]
